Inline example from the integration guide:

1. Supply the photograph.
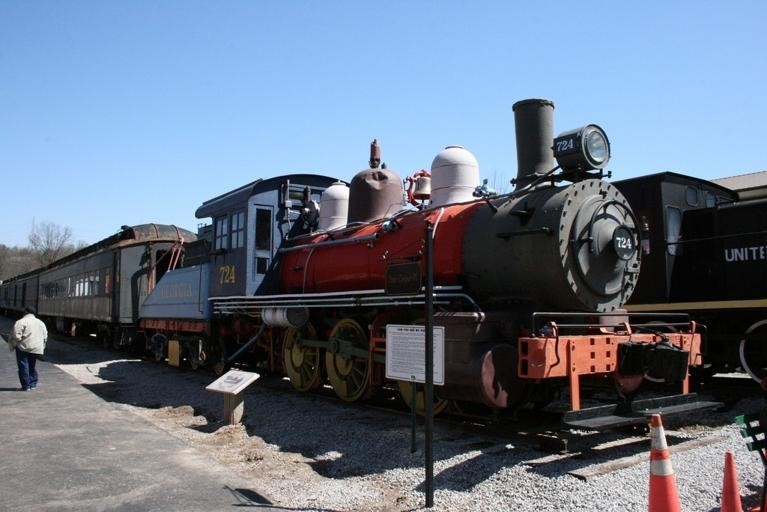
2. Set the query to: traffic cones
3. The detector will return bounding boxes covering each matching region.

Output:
[649,412,745,512]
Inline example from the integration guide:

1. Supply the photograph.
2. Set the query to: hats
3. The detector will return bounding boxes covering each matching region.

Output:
[24,305,36,313]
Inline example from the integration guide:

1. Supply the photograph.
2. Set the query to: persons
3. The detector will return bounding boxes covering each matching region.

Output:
[8,305,48,391]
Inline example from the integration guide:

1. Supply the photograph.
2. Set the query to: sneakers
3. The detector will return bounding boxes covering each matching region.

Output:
[21,388,35,391]
